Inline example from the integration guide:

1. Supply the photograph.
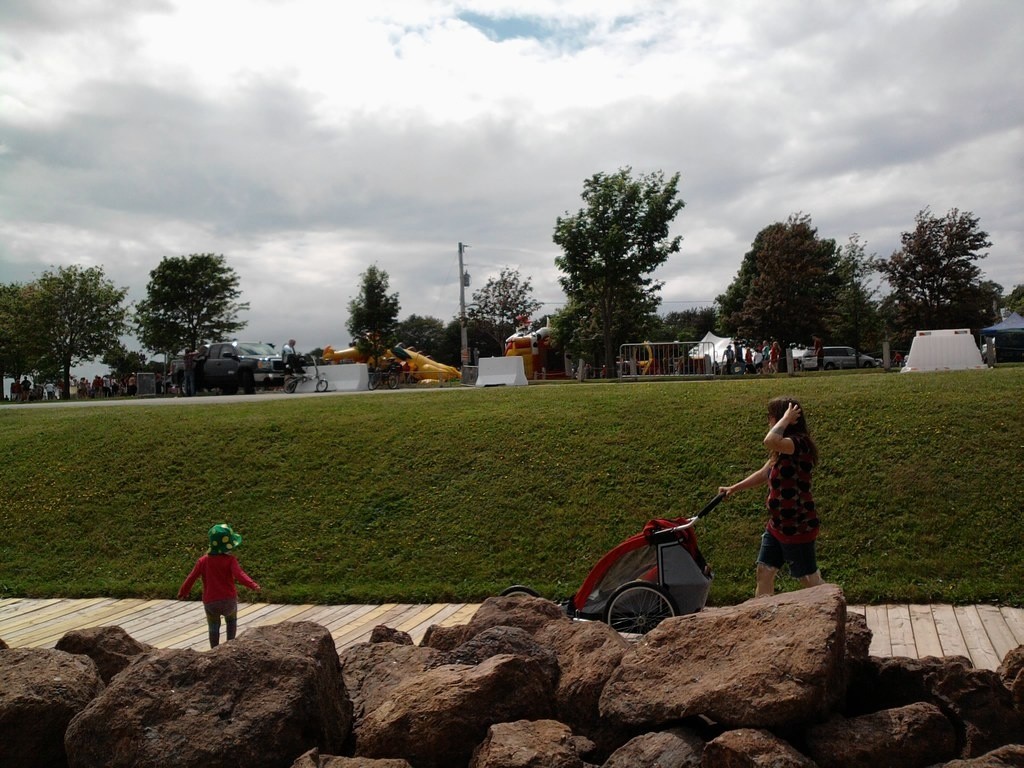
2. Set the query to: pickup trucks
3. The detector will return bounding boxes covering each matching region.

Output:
[170,342,285,397]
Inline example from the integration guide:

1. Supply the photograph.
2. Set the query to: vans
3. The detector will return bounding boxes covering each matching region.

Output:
[801,346,876,370]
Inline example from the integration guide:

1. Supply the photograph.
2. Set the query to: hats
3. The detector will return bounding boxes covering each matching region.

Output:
[206,522,243,554]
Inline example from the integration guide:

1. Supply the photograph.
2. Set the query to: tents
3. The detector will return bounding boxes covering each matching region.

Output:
[688,331,762,368]
[979,311,1024,349]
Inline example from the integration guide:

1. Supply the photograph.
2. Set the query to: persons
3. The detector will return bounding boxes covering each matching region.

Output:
[71,372,137,399]
[570,362,607,379]
[177,523,260,649]
[892,352,903,366]
[721,336,781,375]
[10,377,65,401]
[281,339,301,382]
[811,334,825,372]
[718,399,825,598]
[155,365,182,394]
[183,349,202,397]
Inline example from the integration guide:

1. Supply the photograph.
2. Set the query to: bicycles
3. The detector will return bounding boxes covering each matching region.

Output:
[367,346,402,391]
[283,353,329,394]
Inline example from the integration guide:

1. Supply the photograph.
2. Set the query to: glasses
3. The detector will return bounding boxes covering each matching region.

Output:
[765,412,777,422]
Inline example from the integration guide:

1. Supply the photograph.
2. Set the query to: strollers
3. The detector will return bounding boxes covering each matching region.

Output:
[498,491,729,645]
